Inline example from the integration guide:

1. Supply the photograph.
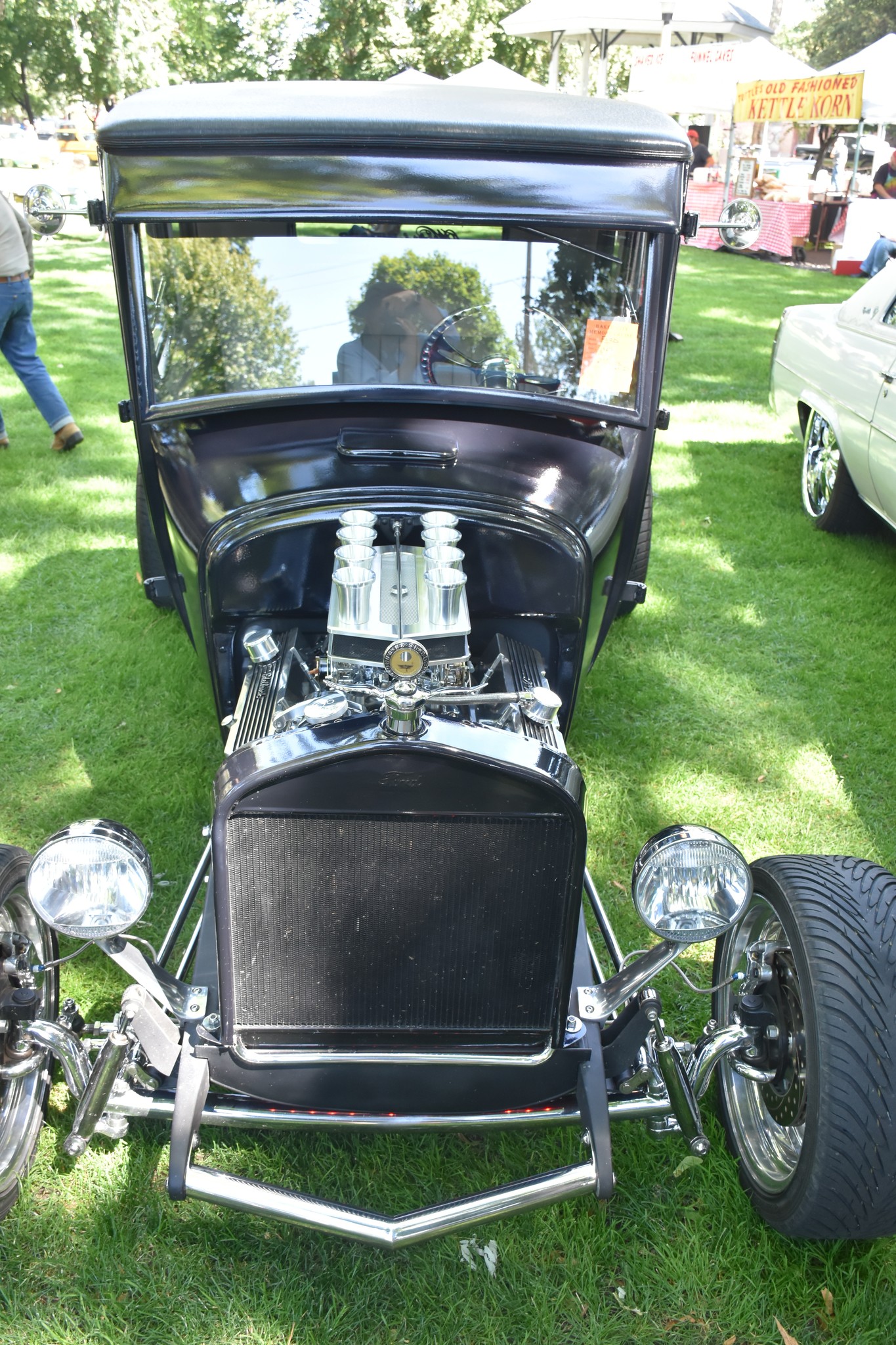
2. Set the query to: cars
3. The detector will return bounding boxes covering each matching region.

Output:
[0,78,896,1259]
[766,260,896,532]
[50,128,98,165]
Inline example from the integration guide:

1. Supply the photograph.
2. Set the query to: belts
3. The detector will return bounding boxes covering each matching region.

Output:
[0,271,29,283]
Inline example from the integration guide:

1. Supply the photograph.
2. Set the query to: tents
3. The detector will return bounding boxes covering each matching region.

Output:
[629,36,818,183]
[722,33,895,200]
[498,0,773,98]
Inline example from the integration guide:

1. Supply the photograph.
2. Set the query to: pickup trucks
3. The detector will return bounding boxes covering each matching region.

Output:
[793,133,896,176]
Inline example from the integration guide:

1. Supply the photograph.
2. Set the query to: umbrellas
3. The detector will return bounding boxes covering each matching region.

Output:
[380,58,554,93]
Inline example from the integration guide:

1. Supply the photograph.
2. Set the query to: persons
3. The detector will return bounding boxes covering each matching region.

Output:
[0,189,85,454]
[870,151,896,199]
[687,129,714,177]
[850,238,896,279]
[830,137,849,193]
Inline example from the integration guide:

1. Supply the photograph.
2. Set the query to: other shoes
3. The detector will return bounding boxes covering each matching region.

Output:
[50,423,84,452]
[0,438,10,448]
[853,272,869,277]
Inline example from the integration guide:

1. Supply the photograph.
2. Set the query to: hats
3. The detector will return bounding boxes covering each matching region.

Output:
[687,129,699,138]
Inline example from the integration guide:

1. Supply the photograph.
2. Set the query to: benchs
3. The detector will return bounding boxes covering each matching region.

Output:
[681,180,813,263]
[331,291,579,387]
